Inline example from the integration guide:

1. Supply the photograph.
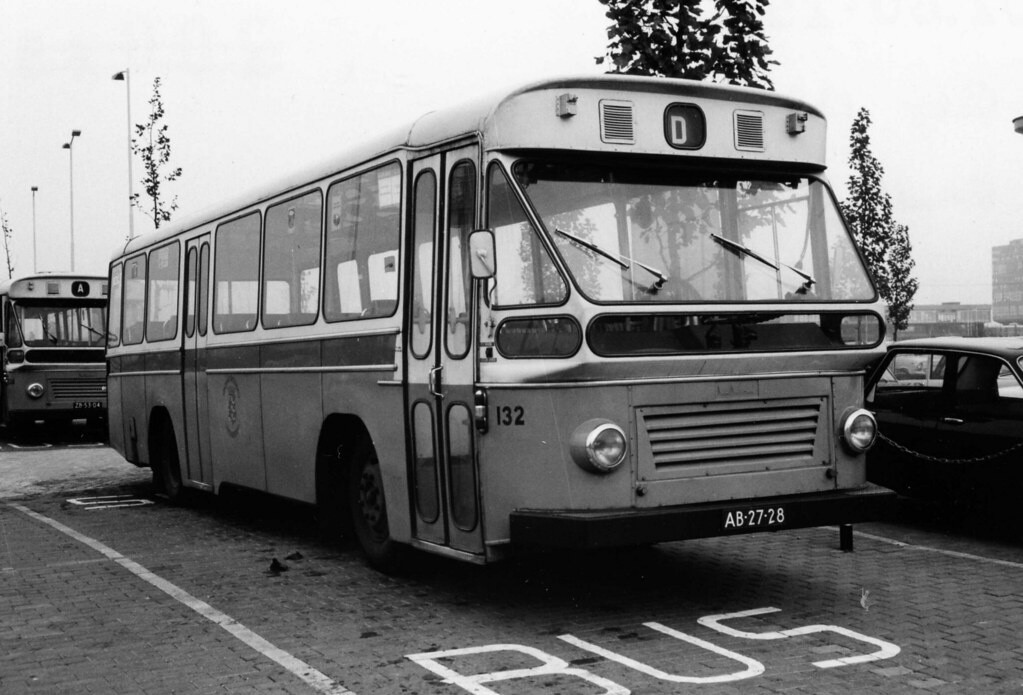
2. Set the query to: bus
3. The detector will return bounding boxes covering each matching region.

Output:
[108,72,891,568]
[0,271,149,443]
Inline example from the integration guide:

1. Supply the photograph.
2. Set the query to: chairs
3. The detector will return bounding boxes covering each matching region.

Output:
[648,276,703,300]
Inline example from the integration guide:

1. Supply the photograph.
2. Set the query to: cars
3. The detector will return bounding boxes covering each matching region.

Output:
[863,333,1022,550]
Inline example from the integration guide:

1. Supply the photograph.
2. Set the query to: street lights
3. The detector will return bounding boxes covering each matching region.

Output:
[63,128,81,273]
[31,185,38,272]
[110,67,133,240]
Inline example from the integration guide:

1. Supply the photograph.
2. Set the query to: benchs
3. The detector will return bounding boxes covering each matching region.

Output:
[124,297,396,344]
